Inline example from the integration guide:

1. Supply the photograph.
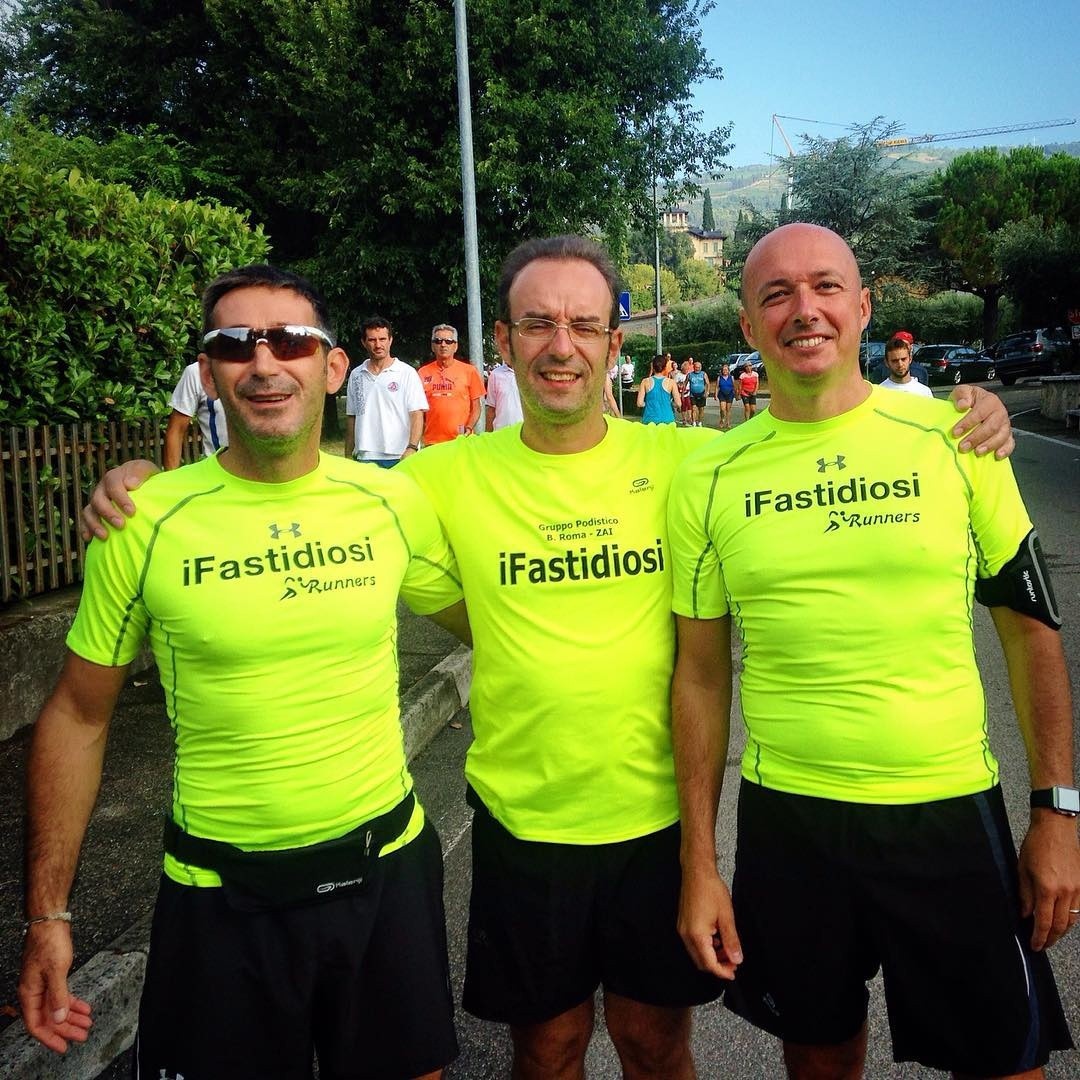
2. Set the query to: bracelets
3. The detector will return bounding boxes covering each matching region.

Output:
[407,444,418,451]
[466,427,472,431]
[20,912,72,936]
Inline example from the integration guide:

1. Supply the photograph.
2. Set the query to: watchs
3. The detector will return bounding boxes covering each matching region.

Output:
[1030,784,1080,817]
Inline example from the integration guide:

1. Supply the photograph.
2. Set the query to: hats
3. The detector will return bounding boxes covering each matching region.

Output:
[744,361,752,367]
[894,332,913,344]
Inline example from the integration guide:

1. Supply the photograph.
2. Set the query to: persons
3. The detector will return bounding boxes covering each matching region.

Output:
[602,354,634,418]
[714,361,759,429]
[484,364,524,433]
[414,323,487,450]
[79,235,1016,1079]
[664,220,1080,1080]
[18,264,473,1079]
[344,318,430,469]
[636,352,710,428]
[164,362,229,471]
[871,331,933,398]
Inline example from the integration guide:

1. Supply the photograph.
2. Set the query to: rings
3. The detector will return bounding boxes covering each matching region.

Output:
[1069,909,1080,913]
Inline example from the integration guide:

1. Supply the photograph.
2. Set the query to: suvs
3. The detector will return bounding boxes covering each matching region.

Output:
[991,326,1080,385]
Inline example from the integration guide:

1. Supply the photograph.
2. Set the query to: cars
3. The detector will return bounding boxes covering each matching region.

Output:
[741,351,768,382]
[859,340,924,375]
[914,344,996,385]
[707,353,751,377]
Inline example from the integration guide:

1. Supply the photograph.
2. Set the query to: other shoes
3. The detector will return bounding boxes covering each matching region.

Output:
[720,422,724,429]
[726,424,731,428]
[683,419,703,426]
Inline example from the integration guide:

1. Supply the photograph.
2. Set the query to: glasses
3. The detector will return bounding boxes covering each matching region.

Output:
[508,318,610,342]
[431,336,457,345]
[199,324,334,361]
